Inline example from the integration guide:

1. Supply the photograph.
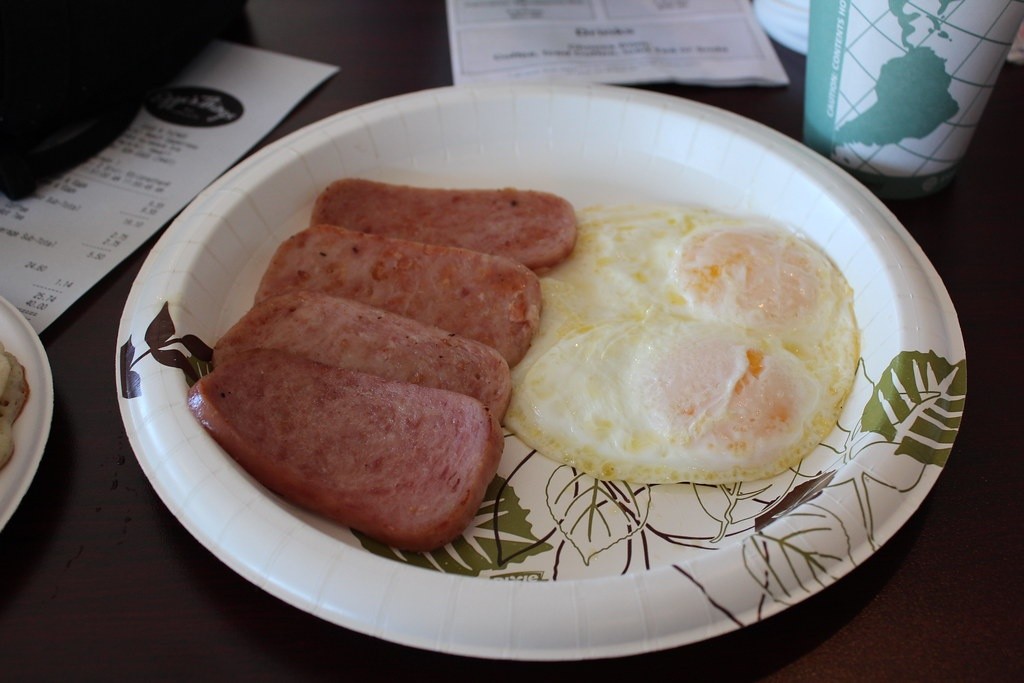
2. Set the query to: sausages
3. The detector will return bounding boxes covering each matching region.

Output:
[188,178,576,551]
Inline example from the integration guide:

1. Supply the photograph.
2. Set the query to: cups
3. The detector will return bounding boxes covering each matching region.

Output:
[790,1,1022,200]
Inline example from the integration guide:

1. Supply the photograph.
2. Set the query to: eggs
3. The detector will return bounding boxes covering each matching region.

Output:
[496,310,858,486]
[547,201,864,364]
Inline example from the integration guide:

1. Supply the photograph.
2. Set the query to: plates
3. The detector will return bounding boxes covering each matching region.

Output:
[0,294,53,553]
[106,81,972,662]
